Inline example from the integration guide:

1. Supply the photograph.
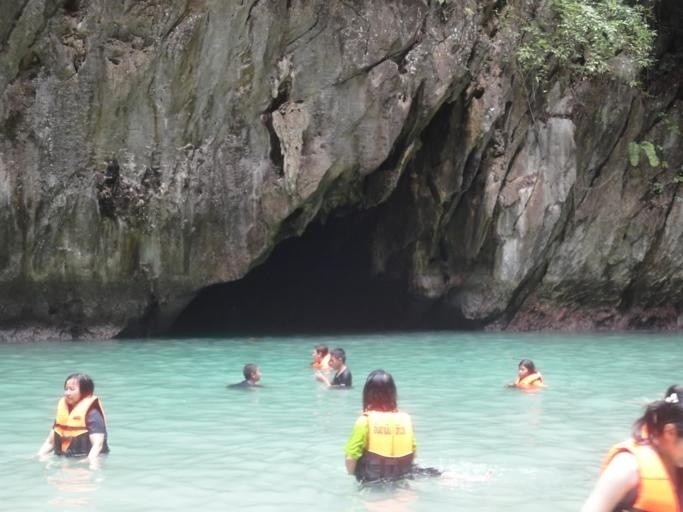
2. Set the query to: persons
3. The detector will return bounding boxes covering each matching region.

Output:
[308,344,333,371]
[580,384,682,512]
[229,362,264,387]
[343,369,442,512]
[506,356,549,390]
[313,348,351,388]
[23,372,109,465]
